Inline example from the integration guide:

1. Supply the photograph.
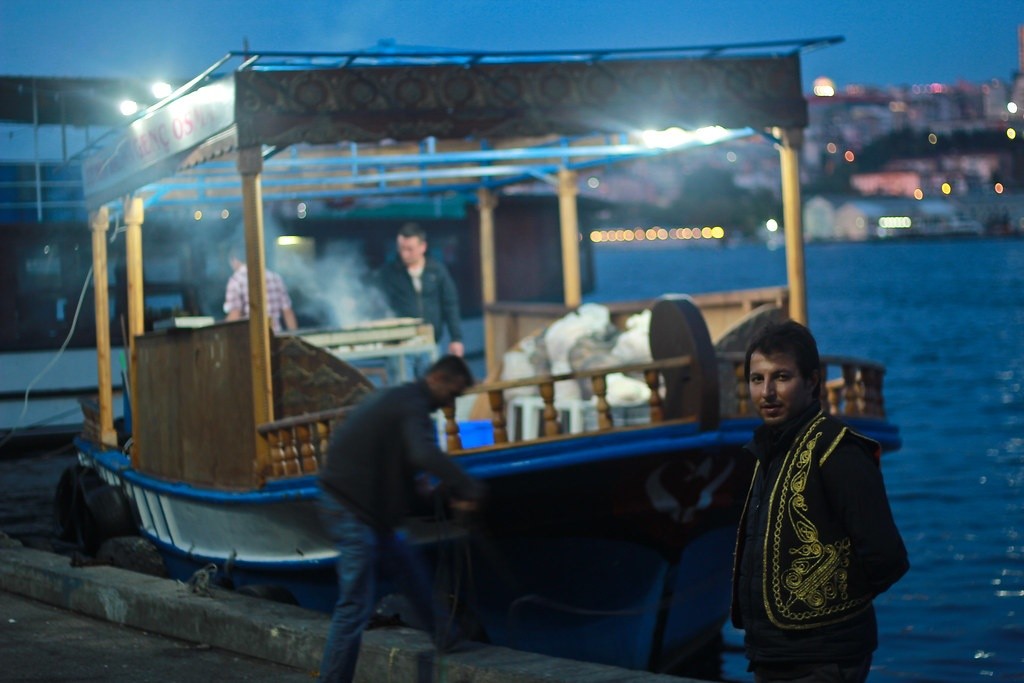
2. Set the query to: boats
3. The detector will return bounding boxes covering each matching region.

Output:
[51,34,888,677]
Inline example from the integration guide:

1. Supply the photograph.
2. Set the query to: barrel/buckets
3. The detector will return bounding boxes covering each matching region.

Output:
[458,420,494,449]
[554,400,600,436]
[605,399,652,426]
[505,402,547,442]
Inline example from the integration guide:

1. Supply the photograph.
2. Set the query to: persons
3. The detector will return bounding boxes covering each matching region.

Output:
[318,355,482,683]
[364,219,467,383]
[730,322,910,683]
[222,248,298,337]
